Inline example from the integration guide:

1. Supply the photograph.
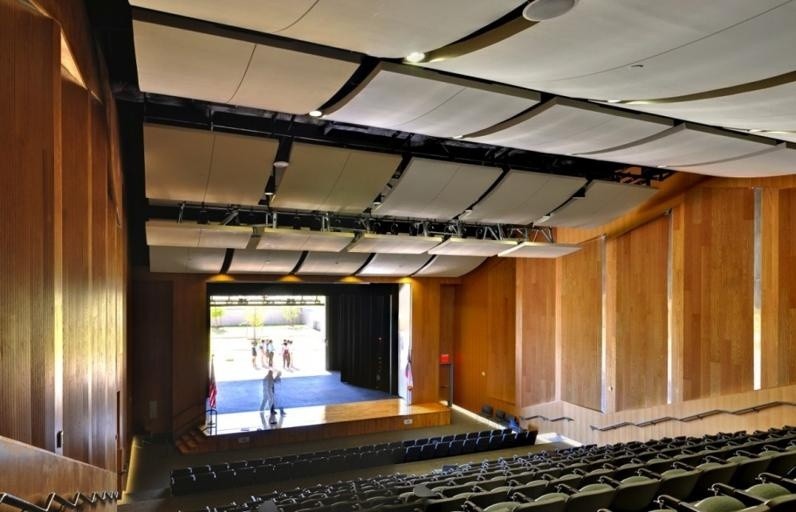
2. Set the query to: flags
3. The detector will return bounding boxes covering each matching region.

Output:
[404,351,413,391]
[208,359,217,410]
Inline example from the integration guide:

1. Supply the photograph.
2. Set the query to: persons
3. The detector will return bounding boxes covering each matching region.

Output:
[259,371,274,415]
[269,371,286,416]
[251,338,292,370]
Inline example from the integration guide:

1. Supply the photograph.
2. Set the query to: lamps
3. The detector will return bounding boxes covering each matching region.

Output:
[257,138,292,208]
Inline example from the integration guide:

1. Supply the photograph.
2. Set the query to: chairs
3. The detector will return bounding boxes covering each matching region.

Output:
[170,425,796,512]
[477,404,518,426]
